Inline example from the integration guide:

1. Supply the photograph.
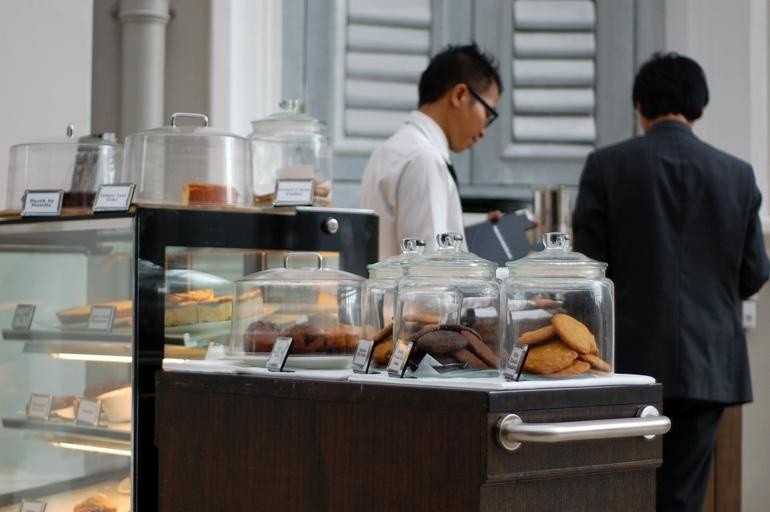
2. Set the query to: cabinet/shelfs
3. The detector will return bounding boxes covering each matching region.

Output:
[153,368,673,512]
[0,205,377,512]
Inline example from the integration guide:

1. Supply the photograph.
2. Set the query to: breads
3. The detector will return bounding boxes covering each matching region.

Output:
[73,493,117,511]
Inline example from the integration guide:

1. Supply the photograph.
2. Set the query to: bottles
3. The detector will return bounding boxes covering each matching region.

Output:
[497,231,619,382]
[358,237,433,376]
[246,100,335,210]
[390,231,501,378]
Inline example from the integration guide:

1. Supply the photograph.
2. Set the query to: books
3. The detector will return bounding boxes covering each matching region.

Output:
[530,184,571,245]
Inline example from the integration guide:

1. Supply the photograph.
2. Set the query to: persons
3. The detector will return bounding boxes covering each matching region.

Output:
[358,43,505,263]
[573,52,769,511]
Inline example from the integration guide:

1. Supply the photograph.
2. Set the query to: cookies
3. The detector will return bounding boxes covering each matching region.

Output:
[517,314,612,380]
[370,313,439,366]
[407,323,499,374]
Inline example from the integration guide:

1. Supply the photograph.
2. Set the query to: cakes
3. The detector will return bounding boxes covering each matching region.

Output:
[25,379,133,425]
[240,320,365,357]
[90,499,93,500]
[164,288,264,329]
[56,301,132,326]
[181,183,238,207]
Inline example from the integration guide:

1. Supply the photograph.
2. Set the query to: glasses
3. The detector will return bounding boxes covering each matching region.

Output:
[467,85,498,127]
